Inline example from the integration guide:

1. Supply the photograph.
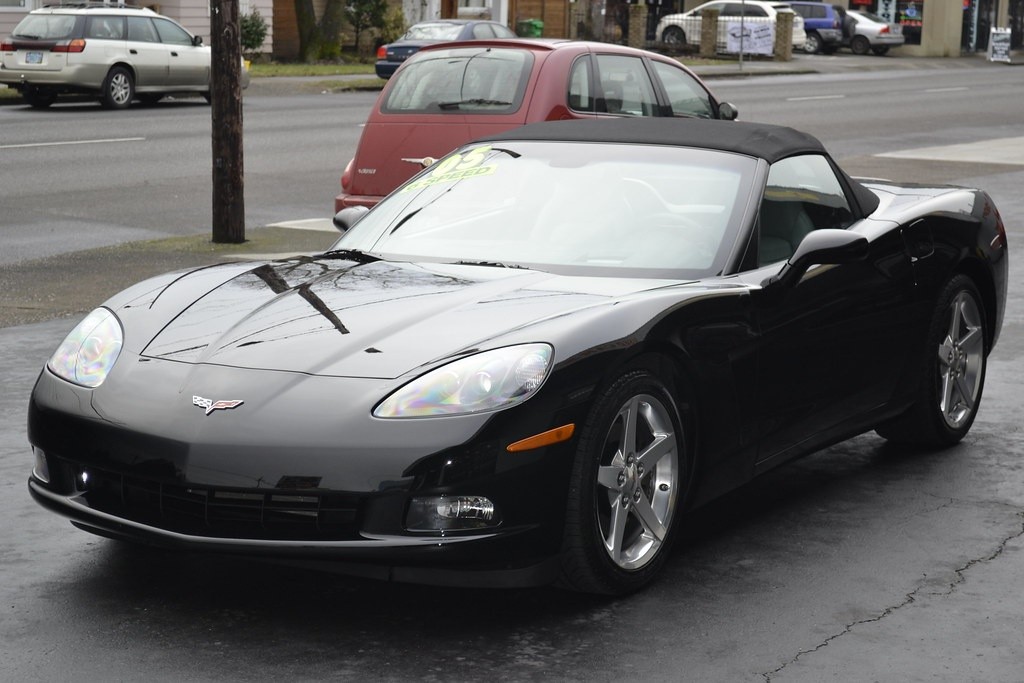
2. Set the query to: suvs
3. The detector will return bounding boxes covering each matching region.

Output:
[3,0,252,110]
[653,0,806,57]
[786,2,855,55]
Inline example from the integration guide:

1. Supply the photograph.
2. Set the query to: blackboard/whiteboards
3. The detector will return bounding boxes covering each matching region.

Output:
[987,31,1011,62]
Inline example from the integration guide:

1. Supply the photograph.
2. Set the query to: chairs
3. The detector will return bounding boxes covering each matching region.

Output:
[600,81,637,115]
[526,173,817,276]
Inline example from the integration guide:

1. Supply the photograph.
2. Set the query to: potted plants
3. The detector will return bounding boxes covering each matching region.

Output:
[241,13,269,73]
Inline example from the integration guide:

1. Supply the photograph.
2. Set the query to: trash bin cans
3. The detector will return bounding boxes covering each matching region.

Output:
[514,20,544,37]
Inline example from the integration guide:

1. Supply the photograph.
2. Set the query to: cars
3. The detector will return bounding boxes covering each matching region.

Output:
[375,17,520,80]
[843,9,906,56]
[331,41,740,240]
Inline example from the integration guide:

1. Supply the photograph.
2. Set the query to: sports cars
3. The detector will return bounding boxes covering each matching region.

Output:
[21,112,1011,598]
